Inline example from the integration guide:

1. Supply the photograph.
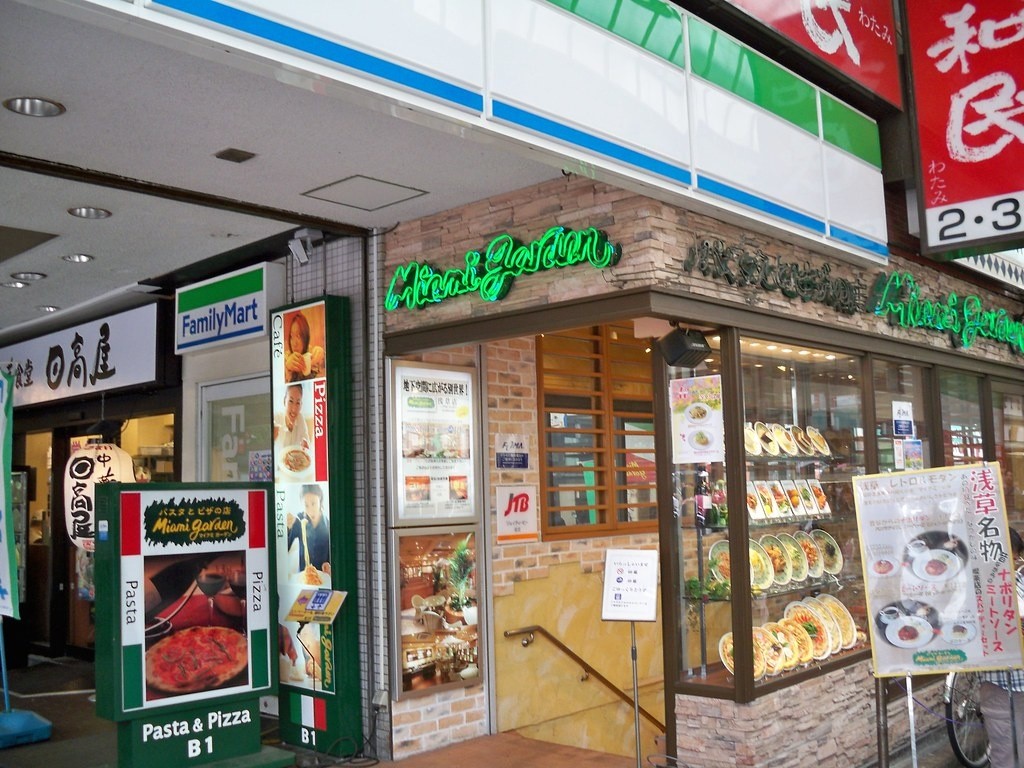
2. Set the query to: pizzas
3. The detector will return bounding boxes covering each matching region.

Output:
[723,597,855,678]
[148,627,248,692]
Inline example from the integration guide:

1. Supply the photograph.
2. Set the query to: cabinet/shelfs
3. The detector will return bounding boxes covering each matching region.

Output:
[681,453,845,680]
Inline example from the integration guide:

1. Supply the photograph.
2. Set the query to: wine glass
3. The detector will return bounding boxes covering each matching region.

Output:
[227,571,247,640]
[194,568,226,626]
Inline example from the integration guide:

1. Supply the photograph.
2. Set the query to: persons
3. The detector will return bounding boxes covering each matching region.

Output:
[287,485,331,574]
[273,385,311,449]
[284,313,324,383]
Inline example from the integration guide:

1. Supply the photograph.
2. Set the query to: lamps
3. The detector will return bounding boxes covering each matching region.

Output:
[655,318,712,368]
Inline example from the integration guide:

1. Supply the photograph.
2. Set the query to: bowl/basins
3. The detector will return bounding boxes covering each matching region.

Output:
[908,540,926,553]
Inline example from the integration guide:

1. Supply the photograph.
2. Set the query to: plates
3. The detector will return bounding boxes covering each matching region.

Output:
[709,528,844,591]
[867,555,899,577]
[912,549,961,583]
[684,402,713,424]
[790,425,814,456]
[907,546,930,558]
[719,593,858,681]
[772,423,798,455]
[745,478,832,519]
[687,429,714,450]
[744,425,762,456]
[291,569,331,590]
[940,623,977,645]
[277,445,314,477]
[880,611,905,624]
[884,616,933,649]
[754,422,780,455]
[806,426,830,456]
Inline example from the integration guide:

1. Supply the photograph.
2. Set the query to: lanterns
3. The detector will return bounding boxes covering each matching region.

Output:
[63,438,135,557]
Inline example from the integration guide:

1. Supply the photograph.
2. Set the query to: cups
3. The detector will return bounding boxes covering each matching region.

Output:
[879,606,899,619]
[301,352,311,376]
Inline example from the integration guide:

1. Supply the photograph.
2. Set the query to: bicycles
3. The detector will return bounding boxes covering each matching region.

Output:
[942,566,1024,768]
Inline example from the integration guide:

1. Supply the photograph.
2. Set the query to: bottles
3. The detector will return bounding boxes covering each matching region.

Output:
[694,466,710,528]
